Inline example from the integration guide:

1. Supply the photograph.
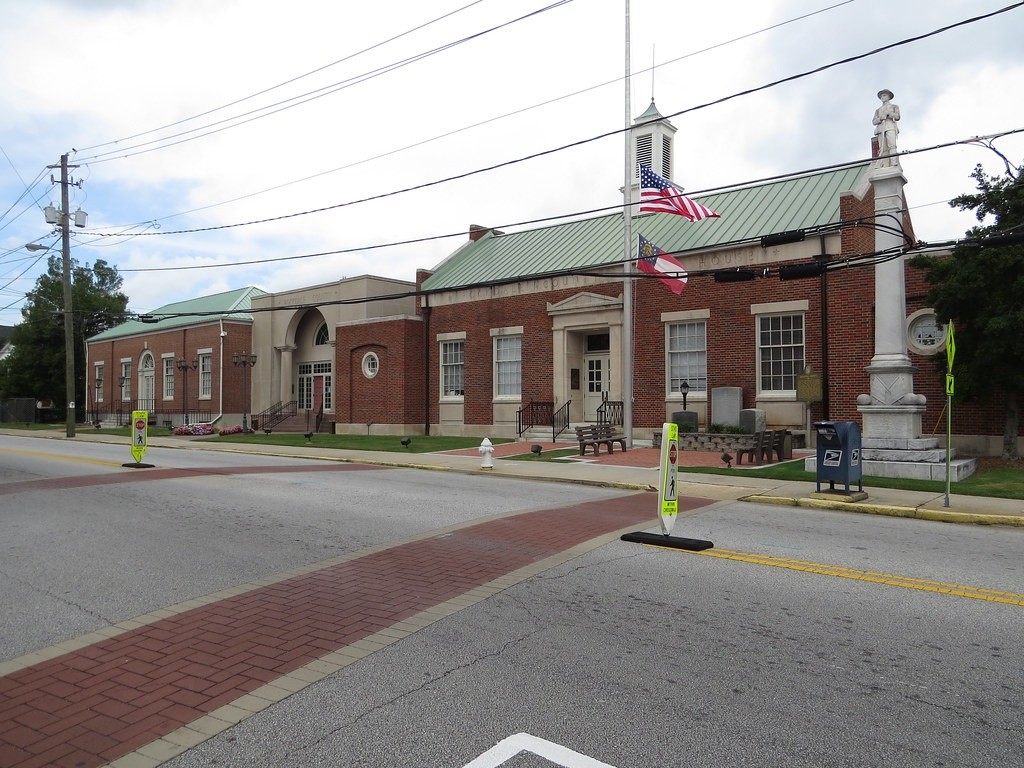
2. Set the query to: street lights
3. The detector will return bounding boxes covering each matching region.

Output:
[94,377,103,430]
[176,355,198,427]
[117,376,128,427]
[22,244,77,440]
[233,350,258,434]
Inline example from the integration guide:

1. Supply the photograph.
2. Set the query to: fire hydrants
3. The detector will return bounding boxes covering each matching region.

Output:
[479,436,496,470]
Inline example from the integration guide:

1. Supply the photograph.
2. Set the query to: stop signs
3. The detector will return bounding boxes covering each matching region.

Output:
[137,421,146,430]
[668,444,679,464]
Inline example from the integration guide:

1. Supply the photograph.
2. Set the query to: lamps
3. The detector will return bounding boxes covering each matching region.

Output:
[721,453,733,467]
[95,424,101,430]
[169,425,175,431]
[530,445,542,456]
[679,381,689,394]
[264,428,272,435]
[304,431,313,441]
[26,422,29,426]
[401,437,411,448]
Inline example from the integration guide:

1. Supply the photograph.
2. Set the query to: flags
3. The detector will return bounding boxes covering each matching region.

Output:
[637,234,688,295]
[639,165,721,223]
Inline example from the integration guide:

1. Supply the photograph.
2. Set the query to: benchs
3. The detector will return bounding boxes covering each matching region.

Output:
[736,429,786,465]
[575,423,628,457]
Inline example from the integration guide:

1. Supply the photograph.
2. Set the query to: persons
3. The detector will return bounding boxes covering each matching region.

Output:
[872,89,901,167]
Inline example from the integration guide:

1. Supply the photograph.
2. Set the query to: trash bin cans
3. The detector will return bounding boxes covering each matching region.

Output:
[812,420,862,496]
